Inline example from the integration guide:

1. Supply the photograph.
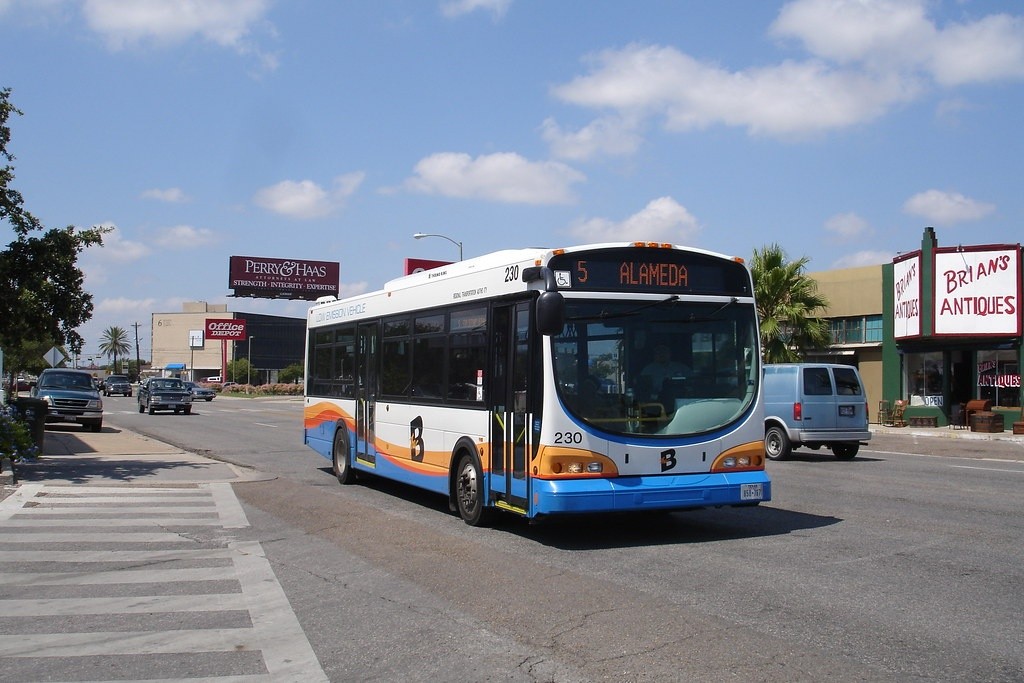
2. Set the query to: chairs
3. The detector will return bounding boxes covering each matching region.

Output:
[877,399,909,427]
[948,399,966,430]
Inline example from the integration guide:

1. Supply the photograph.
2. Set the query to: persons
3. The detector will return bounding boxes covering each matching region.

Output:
[908,364,944,395]
[634,341,695,403]
[173,382,177,387]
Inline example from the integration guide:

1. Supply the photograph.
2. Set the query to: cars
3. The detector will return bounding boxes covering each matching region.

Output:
[184,378,217,403]
[13,373,105,390]
[137,375,192,417]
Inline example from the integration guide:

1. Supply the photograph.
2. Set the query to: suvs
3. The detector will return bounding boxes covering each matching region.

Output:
[102,374,133,399]
[28,367,104,434]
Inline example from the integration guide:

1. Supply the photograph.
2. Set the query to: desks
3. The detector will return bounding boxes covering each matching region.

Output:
[909,416,938,428]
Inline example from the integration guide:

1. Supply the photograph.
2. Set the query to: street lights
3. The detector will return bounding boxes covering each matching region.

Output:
[246,335,255,384]
[412,232,465,263]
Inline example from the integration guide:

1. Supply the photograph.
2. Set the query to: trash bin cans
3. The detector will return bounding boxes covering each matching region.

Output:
[10,397,47,456]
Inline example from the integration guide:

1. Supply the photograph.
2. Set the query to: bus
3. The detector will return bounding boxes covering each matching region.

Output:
[303,240,773,526]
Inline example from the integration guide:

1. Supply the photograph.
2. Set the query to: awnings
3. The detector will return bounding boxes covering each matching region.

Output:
[166,363,186,372]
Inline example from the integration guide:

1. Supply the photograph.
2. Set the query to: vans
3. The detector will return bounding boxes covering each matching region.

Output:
[731,362,875,460]
